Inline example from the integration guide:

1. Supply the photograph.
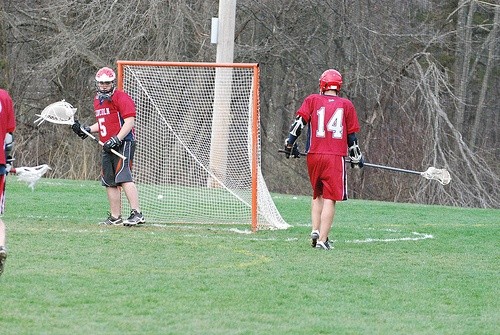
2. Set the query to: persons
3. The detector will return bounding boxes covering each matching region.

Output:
[72,67,145,226]
[0,89,16,275]
[284,69,364,250]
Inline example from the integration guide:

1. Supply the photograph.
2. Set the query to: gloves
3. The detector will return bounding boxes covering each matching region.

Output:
[103,136,122,154]
[71,120,91,140]
[284,138,300,158]
[349,157,363,169]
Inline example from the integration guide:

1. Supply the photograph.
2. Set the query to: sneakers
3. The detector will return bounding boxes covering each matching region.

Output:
[98,211,123,226]
[123,209,145,226]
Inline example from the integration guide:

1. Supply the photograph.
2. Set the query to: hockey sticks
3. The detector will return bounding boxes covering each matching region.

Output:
[277,148,453,186]
[8,163,53,186]
[39,99,128,162]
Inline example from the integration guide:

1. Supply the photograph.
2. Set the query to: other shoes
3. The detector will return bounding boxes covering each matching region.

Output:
[0,246,8,276]
[315,237,334,250]
[310,230,320,248]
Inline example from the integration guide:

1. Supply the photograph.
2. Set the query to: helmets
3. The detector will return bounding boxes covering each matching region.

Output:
[95,67,116,99]
[318,69,342,95]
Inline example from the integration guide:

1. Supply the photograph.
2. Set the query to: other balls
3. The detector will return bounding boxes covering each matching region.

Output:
[158,194,164,200]
